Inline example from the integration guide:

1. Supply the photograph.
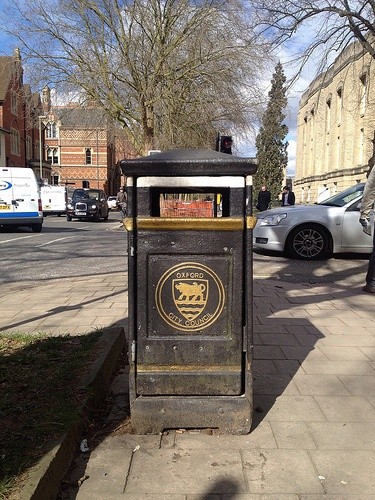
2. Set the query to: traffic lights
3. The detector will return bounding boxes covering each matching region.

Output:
[84,147,92,166]
[221,136,233,155]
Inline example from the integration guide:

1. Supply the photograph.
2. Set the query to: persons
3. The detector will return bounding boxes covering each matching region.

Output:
[256,185,271,212]
[359,164,375,293]
[282,186,295,207]
[116,187,127,211]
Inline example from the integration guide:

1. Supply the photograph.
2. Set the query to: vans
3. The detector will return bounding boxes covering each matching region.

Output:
[0,166,44,233]
[40,185,70,217]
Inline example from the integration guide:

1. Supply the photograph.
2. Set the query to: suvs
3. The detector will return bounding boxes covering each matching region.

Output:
[66,187,109,222]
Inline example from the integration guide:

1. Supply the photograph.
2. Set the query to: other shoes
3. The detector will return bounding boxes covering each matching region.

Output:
[362,285,375,293]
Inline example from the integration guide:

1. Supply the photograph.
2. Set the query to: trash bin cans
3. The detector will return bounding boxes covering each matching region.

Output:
[119,148,260,437]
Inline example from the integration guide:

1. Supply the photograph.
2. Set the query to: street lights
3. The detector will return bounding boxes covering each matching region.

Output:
[38,115,47,179]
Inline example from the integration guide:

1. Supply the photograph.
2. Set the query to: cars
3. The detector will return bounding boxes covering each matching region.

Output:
[107,195,119,212]
[252,181,374,261]
